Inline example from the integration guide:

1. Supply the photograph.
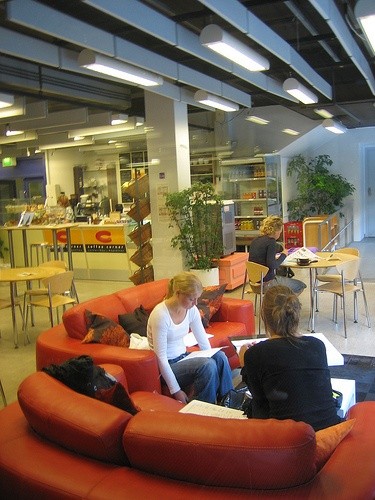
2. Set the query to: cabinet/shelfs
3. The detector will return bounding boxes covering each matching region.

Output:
[218,156,282,247]
[72,165,118,211]
[190,151,216,184]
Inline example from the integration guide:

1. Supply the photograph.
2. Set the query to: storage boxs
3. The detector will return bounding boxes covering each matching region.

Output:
[285,236,303,249]
[284,221,302,236]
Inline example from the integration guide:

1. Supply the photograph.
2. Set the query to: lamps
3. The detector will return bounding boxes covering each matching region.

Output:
[78,48,164,86]
[194,90,240,113]
[135,116,144,126]
[200,23,271,72]
[320,117,348,135]
[282,76,318,105]
[354,0,375,59]
[111,113,129,126]
[0,92,15,109]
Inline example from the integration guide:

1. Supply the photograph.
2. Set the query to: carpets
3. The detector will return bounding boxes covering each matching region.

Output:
[326,354,375,402]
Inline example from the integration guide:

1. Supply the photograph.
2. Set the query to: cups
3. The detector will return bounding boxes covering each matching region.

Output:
[88,216,93,225]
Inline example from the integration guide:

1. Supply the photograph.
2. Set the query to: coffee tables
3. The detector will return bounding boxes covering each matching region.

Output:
[245,377,357,418]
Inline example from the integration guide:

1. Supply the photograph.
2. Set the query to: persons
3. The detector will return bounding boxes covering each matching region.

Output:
[239,285,341,432]
[148,271,233,405]
[248,214,307,297]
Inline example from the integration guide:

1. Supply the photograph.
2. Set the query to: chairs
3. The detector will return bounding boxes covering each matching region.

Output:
[0,228,77,347]
[241,245,372,339]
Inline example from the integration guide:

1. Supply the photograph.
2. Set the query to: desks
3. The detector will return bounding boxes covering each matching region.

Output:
[0,265,67,349]
[279,251,358,334]
[0,222,82,297]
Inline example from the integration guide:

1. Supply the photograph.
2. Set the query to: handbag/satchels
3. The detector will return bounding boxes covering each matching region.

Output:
[222,387,254,418]
[88,367,141,415]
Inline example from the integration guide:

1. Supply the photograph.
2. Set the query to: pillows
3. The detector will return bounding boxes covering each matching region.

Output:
[196,282,228,330]
[314,418,358,471]
[80,308,130,349]
[117,305,151,338]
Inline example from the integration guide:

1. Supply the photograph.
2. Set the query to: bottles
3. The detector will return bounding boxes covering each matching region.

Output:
[254,205,264,215]
[234,219,261,231]
[241,190,275,198]
[286,225,300,246]
[228,166,266,178]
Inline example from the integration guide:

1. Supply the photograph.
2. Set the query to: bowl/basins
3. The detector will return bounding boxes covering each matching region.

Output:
[190,158,210,165]
[297,258,310,266]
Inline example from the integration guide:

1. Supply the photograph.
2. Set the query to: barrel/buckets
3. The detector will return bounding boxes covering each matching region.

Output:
[109,211,121,223]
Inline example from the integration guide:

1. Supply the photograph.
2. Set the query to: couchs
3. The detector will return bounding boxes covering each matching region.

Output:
[35,277,255,392]
[0,361,375,500]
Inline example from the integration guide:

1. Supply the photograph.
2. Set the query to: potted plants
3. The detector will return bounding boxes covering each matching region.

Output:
[162,178,225,286]
